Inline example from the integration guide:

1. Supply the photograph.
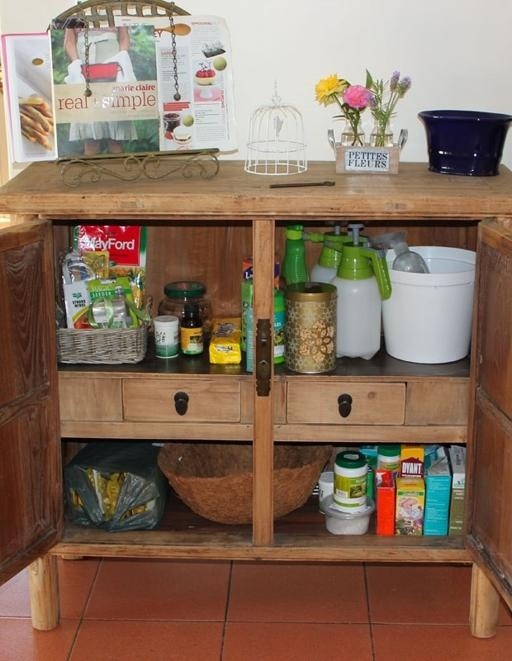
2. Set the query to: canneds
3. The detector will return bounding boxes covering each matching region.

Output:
[157,280,211,339]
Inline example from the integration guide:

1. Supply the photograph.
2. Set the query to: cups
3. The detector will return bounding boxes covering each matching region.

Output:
[162,112,181,140]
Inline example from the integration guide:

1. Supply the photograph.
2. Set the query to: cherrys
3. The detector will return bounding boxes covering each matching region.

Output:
[196,70,214,77]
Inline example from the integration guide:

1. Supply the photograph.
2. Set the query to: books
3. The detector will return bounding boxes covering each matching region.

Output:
[49,22,162,157]
[1,13,240,162]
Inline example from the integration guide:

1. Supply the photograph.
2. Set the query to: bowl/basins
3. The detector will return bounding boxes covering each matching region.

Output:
[193,75,216,85]
[80,60,120,81]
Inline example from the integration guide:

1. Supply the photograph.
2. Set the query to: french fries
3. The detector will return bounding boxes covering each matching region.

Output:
[19,97,54,151]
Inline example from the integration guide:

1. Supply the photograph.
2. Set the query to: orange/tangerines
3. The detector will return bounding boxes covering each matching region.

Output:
[183,114,194,127]
[212,56,227,71]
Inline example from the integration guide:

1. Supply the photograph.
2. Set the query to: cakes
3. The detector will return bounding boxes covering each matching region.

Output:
[173,126,191,141]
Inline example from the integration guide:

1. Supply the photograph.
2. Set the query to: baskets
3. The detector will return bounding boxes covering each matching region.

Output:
[58,323,148,366]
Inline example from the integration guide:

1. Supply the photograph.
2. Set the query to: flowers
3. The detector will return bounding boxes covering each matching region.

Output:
[366,70,412,145]
[316,74,371,146]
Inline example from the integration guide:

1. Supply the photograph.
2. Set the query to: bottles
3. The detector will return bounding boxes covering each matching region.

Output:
[237,255,286,370]
[286,279,339,374]
[311,444,401,535]
[282,223,310,285]
[152,278,216,359]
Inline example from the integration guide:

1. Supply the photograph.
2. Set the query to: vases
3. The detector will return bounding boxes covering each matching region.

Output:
[370,118,394,145]
[342,118,364,146]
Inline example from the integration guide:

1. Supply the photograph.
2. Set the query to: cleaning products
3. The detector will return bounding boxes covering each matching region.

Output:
[372,231,431,274]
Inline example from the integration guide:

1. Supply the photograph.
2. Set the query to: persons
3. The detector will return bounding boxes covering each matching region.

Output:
[65,24,138,154]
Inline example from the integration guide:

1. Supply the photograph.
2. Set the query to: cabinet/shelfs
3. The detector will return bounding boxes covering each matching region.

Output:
[1,162,511,638]
